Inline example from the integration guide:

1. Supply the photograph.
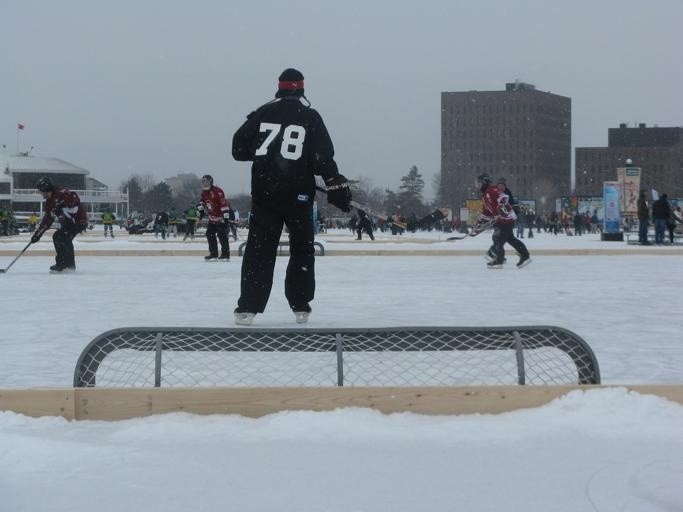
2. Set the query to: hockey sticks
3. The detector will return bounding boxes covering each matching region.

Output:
[448,219,493,241]
[315,185,447,232]
[1,224,48,272]
[189,209,240,225]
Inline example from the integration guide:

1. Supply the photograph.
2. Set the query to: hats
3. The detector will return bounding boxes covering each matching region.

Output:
[274,67,305,100]
[495,177,507,185]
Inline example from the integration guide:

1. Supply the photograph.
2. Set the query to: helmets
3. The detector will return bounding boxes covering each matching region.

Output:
[201,174,213,186]
[476,173,492,185]
[34,177,53,192]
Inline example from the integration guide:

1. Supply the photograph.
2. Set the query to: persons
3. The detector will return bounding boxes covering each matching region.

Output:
[637,190,683,245]
[318,174,601,266]
[231,68,353,316]
[0,175,240,272]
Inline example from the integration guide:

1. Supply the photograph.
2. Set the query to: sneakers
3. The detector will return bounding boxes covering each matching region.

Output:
[49,262,63,270]
[516,254,529,266]
[232,305,258,315]
[203,255,218,259]
[486,259,505,266]
[54,263,76,271]
[218,254,230,260]
[292,301,312,314]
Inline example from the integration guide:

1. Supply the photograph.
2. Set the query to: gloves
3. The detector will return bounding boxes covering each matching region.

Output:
[29,233,39,244]
[197,208,205,221]
[324,172,353,214]
[222,212,230,226]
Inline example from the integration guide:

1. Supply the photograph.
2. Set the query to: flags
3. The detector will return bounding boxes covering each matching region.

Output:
[17,122,24,129]
